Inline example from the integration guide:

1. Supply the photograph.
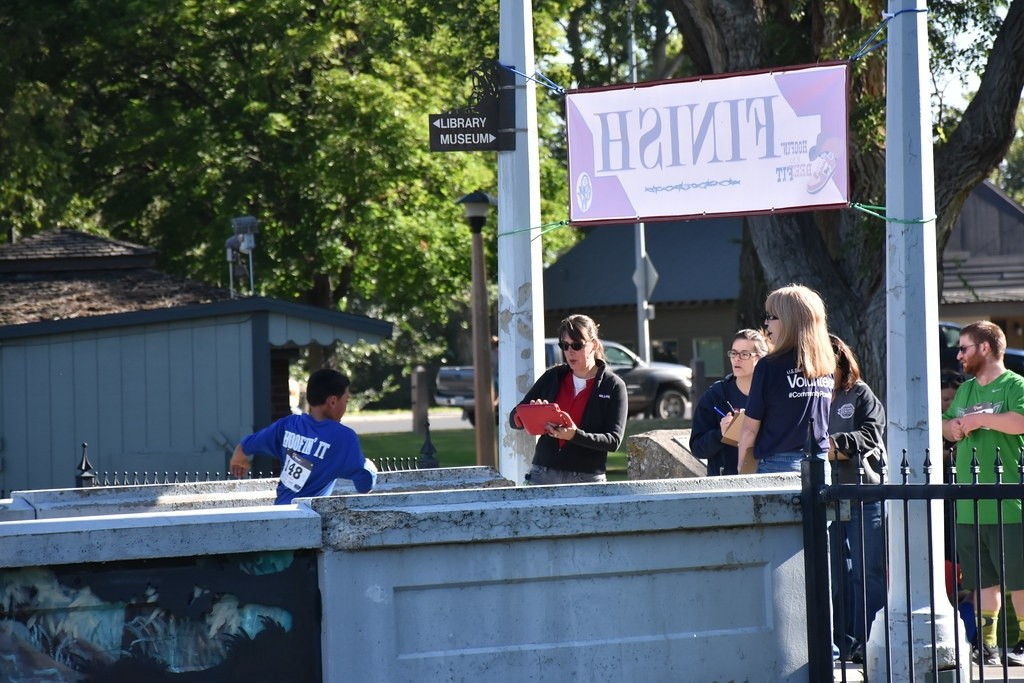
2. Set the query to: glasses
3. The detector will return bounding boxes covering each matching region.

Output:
[558,338,593,351]
[765,311,779,320]
[727,350,759,360]
[959,344,974,354]
[941,374,966,383]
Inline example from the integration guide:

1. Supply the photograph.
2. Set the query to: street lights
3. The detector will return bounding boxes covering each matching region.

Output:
[454,188,499,465]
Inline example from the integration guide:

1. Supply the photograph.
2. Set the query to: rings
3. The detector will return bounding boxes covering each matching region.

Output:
[554,434,558,438]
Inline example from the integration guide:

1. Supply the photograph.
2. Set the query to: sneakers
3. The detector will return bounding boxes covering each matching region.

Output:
[972,643,1002,667]
[1002,640,1024,666]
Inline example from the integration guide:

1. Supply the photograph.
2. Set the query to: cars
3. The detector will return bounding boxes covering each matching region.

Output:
[938,321,1024,391]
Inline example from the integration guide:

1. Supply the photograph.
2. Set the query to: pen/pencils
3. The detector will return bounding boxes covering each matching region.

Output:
[557,424,567,428]
[713,406,726,417]
[726,400,735,415]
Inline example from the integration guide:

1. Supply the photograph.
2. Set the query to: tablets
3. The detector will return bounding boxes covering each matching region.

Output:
[517,403,567,435]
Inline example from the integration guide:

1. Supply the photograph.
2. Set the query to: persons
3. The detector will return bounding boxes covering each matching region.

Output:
[508,314,629,486]
[941,321,1024,667]
[737,286,835,486]
[229,370,378,510]
[826,333,889,664]
[690,329,774,478]
[939,369,984,652]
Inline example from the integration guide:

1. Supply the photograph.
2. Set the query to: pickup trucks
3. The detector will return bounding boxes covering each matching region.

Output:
[435,336,693,427]
